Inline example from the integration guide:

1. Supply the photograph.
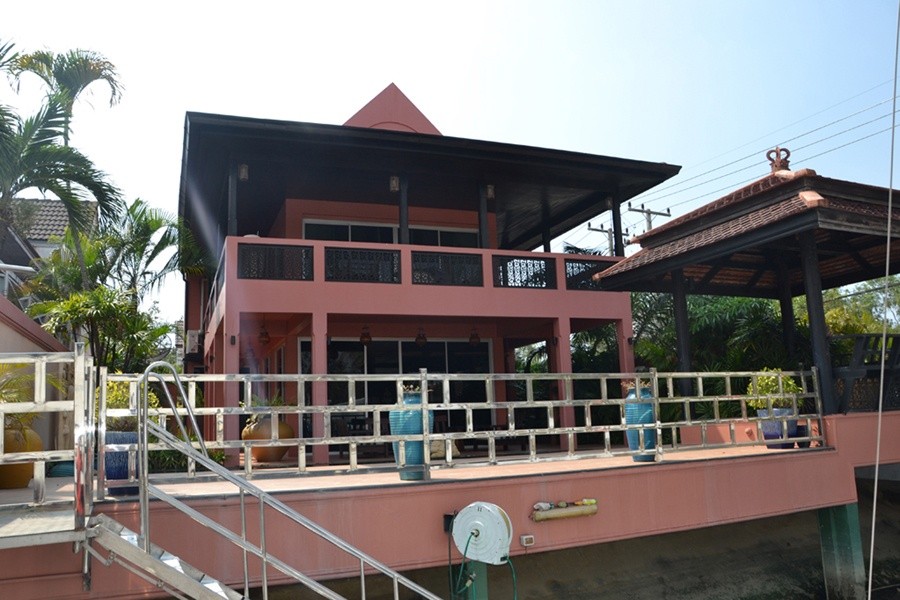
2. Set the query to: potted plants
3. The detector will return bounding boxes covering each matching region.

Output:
[238,388,294,462]
[746,366,804,449]
[389,385,434,480]
[621,379,657,461]
[106,416,143,496]
[0,362,70,489]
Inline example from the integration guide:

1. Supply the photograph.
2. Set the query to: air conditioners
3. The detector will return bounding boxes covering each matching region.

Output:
[185,330,199,355]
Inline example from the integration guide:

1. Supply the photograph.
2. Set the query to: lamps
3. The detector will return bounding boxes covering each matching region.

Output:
[487,180,496,200]
[360,315,371,346]
[415,316,427,348]
[258,313,270,347]
[237,163,249,182]
[469,318,480,348]
[389,175,400,193]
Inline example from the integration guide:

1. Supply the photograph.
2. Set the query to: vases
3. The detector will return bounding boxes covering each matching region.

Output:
[797,426,810,448]
